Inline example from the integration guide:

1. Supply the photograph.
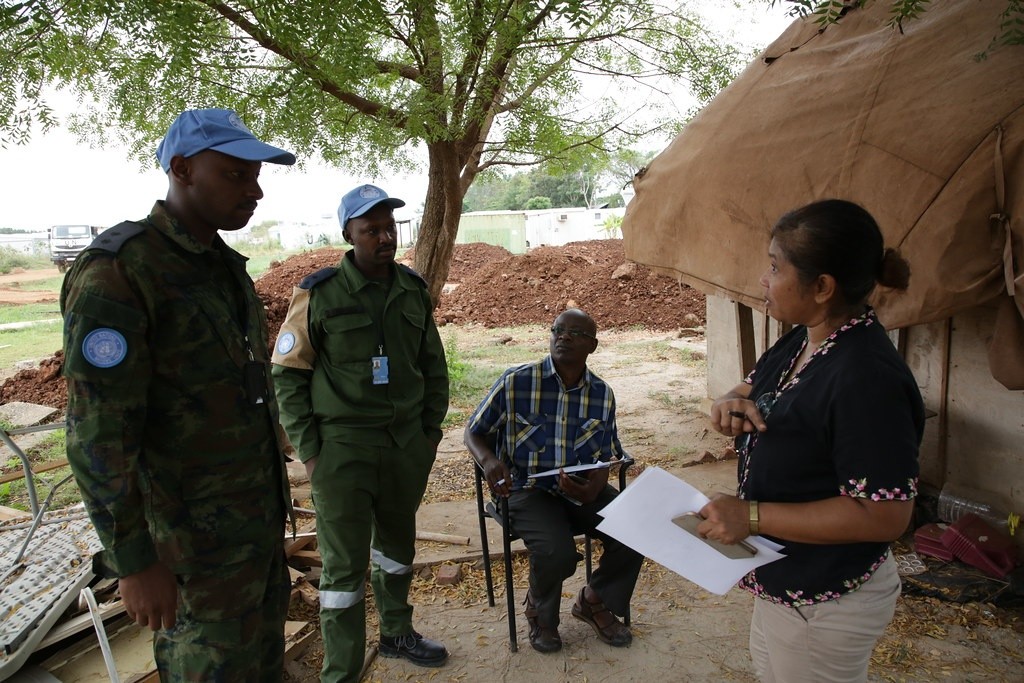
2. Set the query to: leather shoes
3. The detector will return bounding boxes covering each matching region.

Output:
[378,628,449,667]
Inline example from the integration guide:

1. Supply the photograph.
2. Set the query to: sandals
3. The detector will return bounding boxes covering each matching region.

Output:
[522,588,562,652]
[571,586,632,646]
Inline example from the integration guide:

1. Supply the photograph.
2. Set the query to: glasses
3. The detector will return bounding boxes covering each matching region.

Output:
[551,326,596,342]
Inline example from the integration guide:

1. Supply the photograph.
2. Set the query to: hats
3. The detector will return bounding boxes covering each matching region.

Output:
[338,184,405,230]
[156,108,296,174]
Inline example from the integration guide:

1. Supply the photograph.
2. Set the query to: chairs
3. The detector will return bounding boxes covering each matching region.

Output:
[474,448,635,651]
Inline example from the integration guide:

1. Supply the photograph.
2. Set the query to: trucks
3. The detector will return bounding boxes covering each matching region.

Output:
[46,224,101,272]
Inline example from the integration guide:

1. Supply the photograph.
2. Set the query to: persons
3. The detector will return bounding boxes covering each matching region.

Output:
[59,109,298,682]
[372,362,381,371]
[462,308,645,654]
[695,197,925,682]
[268,184,450,683]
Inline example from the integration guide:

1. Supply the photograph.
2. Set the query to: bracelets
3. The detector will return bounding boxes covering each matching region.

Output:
[748,500,758,536]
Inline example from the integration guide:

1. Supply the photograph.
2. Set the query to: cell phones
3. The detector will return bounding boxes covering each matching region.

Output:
[671,511,758,559]
